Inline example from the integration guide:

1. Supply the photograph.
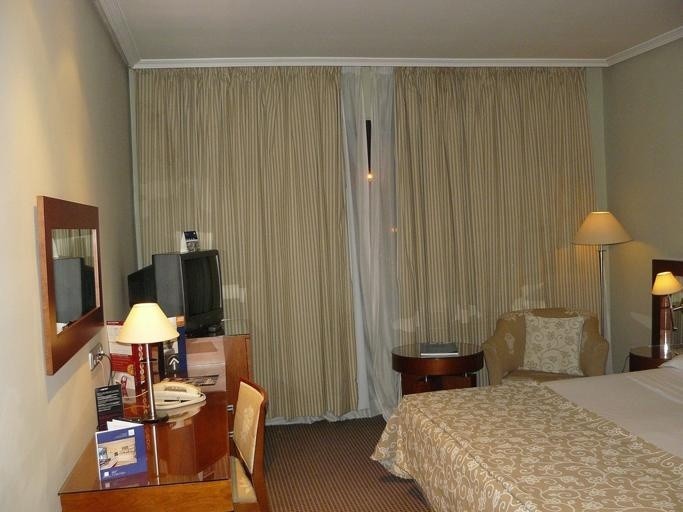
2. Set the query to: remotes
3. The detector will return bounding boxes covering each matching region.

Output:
[208,321,220,333]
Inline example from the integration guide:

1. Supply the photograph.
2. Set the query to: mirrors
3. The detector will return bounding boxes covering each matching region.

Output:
[37,198,105,375]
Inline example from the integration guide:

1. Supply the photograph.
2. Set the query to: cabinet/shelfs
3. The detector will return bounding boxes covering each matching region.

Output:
[182,334,252,386]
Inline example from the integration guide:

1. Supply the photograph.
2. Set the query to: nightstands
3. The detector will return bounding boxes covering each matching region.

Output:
[628,347,672,371]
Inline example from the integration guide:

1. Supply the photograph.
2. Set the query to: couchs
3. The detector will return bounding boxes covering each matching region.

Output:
[483,307,610,385]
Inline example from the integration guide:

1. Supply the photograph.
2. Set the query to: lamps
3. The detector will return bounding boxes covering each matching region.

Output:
[571,211,632,339]
[119,302,179,426]
[650,270,683,331]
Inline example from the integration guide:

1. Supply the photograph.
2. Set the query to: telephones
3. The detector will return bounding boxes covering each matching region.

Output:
[152,382,206,403]
[154,403,204,424]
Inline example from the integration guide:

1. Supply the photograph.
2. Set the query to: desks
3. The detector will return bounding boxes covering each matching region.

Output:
[58,327,254,511]
[391,343,483,392]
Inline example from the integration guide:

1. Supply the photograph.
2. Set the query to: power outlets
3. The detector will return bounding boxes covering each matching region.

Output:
[90,344,105,371]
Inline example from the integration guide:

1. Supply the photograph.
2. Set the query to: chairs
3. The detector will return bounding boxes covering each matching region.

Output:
[231,376,270,511]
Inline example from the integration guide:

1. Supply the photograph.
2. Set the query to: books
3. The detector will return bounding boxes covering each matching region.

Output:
[96,419,146,480]
[419,342,458,357]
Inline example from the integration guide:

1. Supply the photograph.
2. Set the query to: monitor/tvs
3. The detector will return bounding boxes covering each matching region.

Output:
[128,248,223,333]
[53,256,96,324]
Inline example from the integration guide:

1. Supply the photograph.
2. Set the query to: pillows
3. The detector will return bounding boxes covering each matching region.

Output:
[658,354,683,370]
[524,315,584,375]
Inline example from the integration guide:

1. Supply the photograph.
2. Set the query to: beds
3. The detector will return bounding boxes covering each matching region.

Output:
[369,260,681,511]
